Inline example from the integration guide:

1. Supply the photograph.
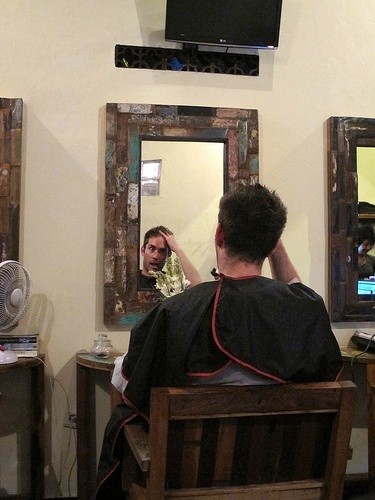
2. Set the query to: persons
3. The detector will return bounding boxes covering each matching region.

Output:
[358,225,375,280]
[138,226,204,302]
[111,185,343,499]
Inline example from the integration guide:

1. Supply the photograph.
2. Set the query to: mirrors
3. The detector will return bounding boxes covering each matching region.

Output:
[327,116,375,323]
[105,102,259,331]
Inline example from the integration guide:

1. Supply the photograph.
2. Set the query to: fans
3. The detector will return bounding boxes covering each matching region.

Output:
[0,260,31,364]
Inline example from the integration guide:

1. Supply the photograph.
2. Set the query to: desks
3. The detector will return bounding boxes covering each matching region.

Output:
[73,351,129,500]
[0,354,47,500]
[342,344,375,499]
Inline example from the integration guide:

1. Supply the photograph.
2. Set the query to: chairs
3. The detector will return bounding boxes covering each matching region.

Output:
[122,379,356,500]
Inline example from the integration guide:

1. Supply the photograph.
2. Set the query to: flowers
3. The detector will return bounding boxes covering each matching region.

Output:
[148,255,192,298]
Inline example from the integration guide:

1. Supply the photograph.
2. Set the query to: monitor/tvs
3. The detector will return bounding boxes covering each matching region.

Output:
[357,280,375,296]
[164,0,283,53]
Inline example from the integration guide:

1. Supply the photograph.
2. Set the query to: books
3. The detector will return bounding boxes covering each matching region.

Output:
[0,334,39,357]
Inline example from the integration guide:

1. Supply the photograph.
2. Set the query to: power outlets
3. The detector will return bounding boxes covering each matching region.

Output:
[63,408,77,429]
